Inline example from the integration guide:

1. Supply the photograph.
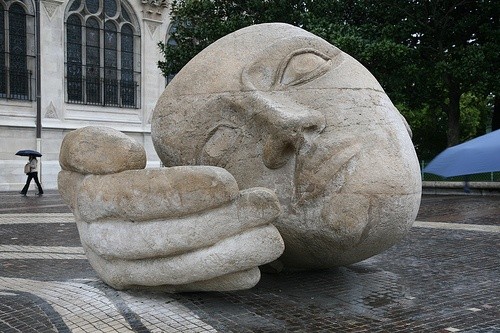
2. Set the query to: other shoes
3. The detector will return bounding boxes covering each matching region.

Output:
[38,190,43,194]
[19,191,26,195]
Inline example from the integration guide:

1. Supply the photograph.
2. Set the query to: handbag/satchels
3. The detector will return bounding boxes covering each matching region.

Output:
[24,163,31,173]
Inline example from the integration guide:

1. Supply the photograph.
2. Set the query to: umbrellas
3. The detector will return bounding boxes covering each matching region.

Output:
[424,126,500,179]
[16,150,42,157]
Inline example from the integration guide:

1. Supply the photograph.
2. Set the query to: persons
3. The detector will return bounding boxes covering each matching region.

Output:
[20,155,44,196]
[56,22,423,293]
